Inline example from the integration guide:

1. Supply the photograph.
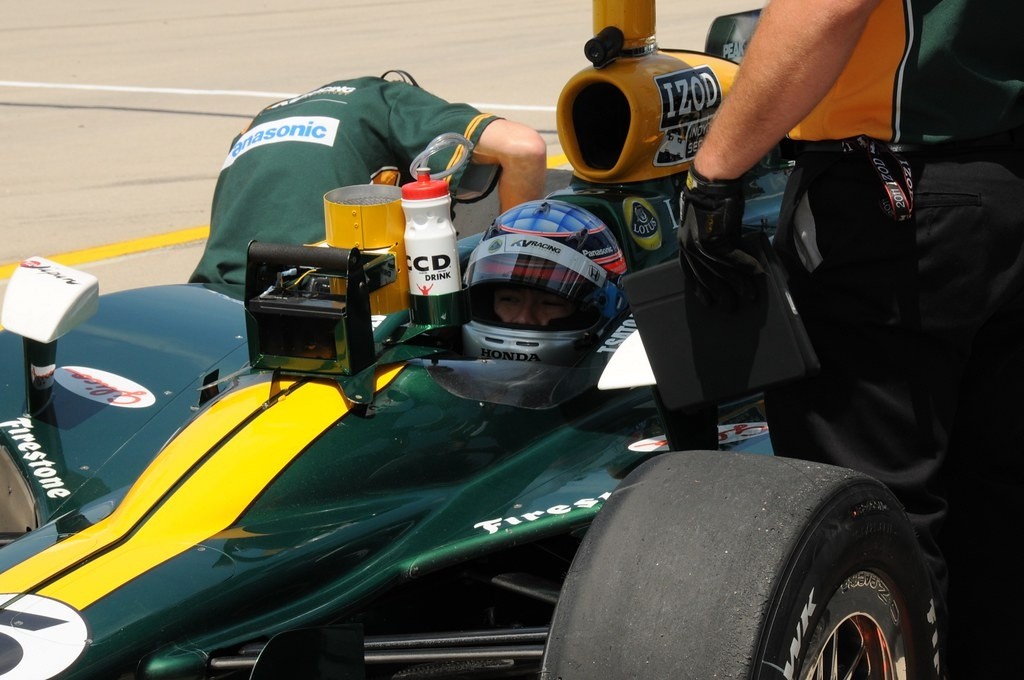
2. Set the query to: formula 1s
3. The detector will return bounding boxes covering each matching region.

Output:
[1,0,944,680]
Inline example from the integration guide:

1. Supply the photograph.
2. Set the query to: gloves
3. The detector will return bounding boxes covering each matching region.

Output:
[681,160,759,306]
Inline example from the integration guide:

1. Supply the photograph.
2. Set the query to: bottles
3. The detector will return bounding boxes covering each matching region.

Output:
[402,168,461,296]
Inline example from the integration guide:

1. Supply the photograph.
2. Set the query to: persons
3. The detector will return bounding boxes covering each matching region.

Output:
[462,199,626,364]
[677,0,1024,679]
[186,76,547,284]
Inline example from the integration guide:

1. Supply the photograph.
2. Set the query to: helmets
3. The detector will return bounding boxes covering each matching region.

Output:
[459,196,628,364]
